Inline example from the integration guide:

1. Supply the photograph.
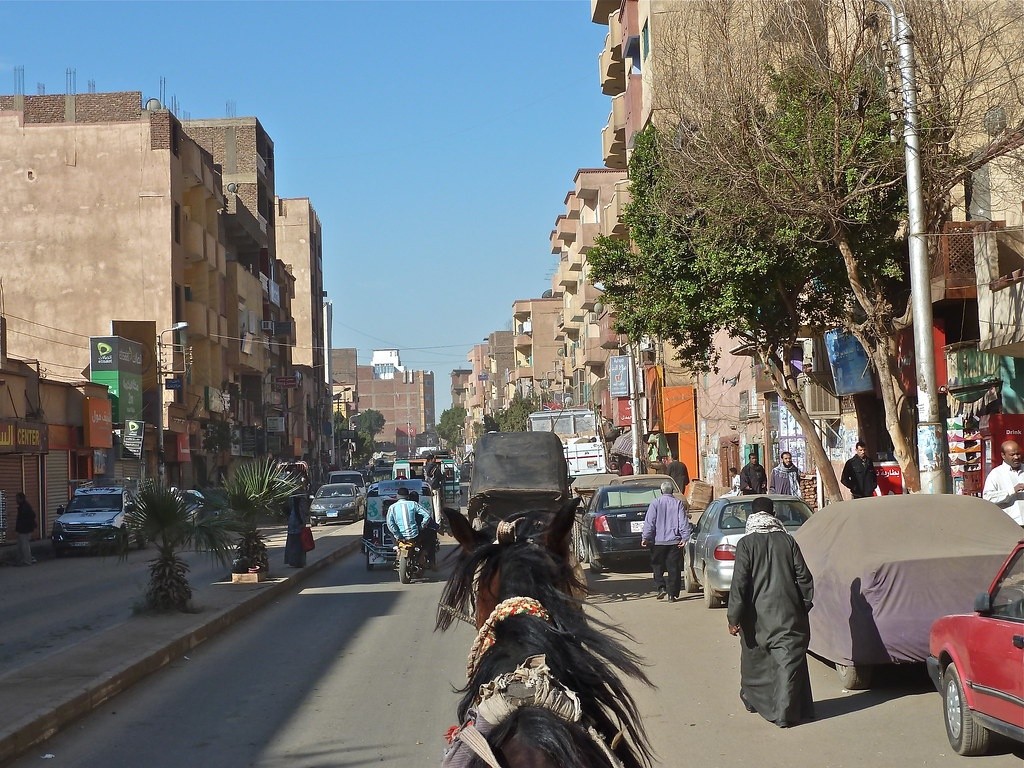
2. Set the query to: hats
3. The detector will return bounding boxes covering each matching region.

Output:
[752,497,774,515]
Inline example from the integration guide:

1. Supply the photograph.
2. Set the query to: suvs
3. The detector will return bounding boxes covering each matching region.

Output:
[51,486,149,558]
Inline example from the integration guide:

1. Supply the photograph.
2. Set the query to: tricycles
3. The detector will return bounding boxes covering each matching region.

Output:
[467,431,572,553]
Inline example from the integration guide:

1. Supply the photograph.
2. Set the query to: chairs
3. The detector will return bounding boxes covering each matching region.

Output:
[775,513,791,524]
[724,516,741,527]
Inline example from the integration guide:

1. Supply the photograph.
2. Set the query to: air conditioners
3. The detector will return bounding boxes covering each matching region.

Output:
[261,321,274,330]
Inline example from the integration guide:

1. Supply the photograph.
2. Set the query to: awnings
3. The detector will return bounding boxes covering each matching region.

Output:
[610,430,633,458]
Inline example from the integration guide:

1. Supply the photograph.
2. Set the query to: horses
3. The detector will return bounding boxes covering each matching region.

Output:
[432,497,663,768]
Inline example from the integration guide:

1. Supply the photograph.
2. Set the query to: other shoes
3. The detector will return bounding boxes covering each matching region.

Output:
[740,689,756,711]
[668,596,677,603]
[657,587,667,599]
[774,718,790,728]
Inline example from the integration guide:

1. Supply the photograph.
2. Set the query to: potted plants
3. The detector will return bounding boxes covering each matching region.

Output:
[225,461,301,582]
[130,485,207,613]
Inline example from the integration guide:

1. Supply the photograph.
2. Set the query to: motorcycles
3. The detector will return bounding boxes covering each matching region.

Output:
[359,447,461,490]
[394,524,440,584]
[361,479,436,571]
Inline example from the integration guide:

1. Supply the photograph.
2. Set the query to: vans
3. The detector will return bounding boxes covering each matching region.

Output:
[325,470,367,499]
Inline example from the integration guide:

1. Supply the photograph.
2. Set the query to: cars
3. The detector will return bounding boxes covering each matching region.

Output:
[309,483,366,527]
[576,482,663,575]
[925,542,1024,756]
[683,494,813,609]
[609,474,690,515]
[169,486,205,526]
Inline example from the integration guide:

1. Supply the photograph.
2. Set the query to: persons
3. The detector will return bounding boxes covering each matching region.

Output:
[640,482,690,602]
[840,442,877,499]
[423,454,448,491]
[15,493,37,567]
[727,497,814,728]
[769,452,802,519]
[285,497,311,568]
[665,454,689,495]
[386,488,438,565]
[719,468,743,497]
[621,458,633,475]
[982,439,1024,529]
[740,453,767,517]
[650,456,667,474]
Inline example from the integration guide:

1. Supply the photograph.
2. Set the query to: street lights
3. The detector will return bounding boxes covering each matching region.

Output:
[337,387,352,470]
[158,321,189,497]
[593,282,640,476]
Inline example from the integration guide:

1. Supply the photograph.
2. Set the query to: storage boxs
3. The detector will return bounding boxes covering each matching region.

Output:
[946,415,983,497]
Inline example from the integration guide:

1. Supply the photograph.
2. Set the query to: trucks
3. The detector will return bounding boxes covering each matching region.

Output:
[529,408,606,485]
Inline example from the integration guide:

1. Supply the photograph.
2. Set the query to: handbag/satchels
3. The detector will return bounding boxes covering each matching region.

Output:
[301,527,315,552]
